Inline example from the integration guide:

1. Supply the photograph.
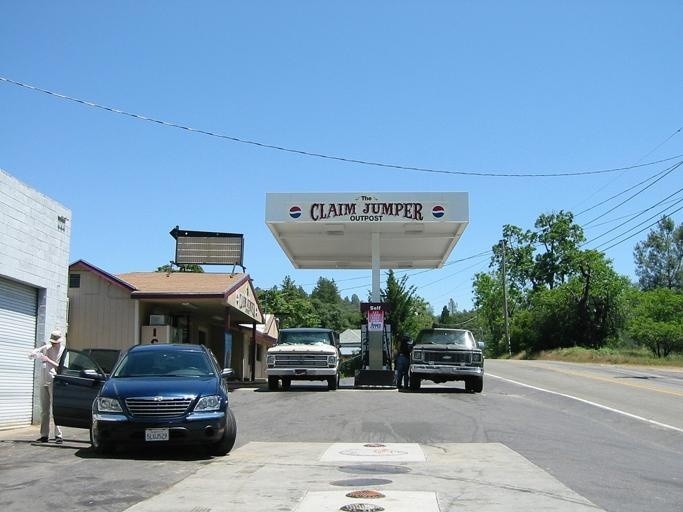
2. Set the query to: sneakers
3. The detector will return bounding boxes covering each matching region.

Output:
[35,437,48,442]
[55,436,62,443]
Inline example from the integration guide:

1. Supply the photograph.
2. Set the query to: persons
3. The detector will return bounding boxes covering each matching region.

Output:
[396,332,416,389]
[31,331,68,444]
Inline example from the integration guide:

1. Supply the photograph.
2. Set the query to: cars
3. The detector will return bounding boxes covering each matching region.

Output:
[52,342,236,455]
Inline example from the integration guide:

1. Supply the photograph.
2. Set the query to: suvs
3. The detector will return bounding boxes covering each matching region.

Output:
[265,328,341,390]
[408,324,484,394]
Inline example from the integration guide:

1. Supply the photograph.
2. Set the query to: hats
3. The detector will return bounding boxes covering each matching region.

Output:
[47,330,63,344]
